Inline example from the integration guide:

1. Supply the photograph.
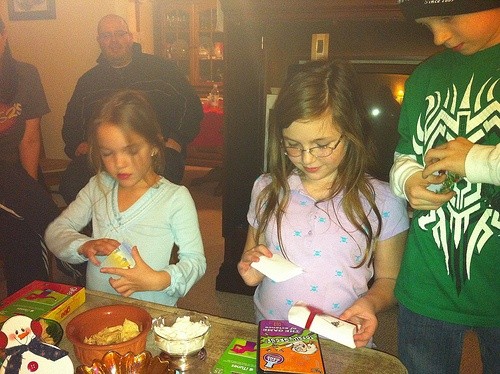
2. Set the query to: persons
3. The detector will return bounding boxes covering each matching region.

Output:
[236,57,411,348]
[390,0,500,374]
[59,14,205,205]
[0,17,52,296]
[44,90,207,306]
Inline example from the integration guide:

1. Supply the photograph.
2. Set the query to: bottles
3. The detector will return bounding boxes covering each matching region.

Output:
[210,84,219,108]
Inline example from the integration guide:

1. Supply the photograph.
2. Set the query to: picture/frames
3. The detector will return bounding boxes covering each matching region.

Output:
[9,0,56,20]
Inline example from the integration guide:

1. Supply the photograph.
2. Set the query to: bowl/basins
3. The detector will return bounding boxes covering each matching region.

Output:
[67,304,151,363]
[153,311,212,358]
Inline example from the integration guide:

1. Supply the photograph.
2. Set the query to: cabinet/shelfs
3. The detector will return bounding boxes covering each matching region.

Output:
[150,0,223,166]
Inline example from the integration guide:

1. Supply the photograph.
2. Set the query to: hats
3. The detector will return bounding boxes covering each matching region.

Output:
[399,0,500,22]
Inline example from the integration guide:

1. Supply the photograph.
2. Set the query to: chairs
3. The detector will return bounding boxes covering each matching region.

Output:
[38,135,71,194]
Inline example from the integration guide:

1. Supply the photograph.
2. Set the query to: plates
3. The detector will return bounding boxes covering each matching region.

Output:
[35,319,63,346]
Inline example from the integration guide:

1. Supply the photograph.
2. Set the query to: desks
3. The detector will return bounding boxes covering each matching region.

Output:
[57,289,409,374]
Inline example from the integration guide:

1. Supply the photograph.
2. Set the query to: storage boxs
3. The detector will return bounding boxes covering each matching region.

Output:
[0,279,86,331]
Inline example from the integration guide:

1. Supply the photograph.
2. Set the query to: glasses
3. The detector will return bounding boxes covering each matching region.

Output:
[279,135,343,157]
[99,31,128,41]
